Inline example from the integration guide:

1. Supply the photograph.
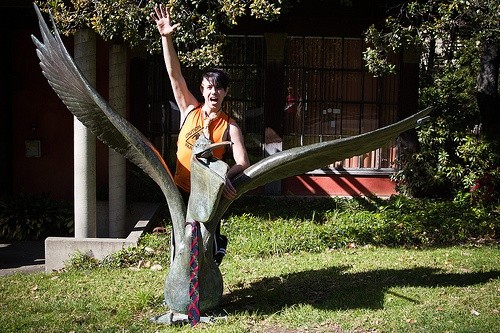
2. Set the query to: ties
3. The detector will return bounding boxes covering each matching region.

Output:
[183,220,201,329]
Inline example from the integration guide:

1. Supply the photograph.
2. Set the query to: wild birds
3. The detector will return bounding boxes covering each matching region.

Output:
[31,2,432,326]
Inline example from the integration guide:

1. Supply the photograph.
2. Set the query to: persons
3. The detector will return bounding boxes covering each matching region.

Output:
[151,3,250,264]
